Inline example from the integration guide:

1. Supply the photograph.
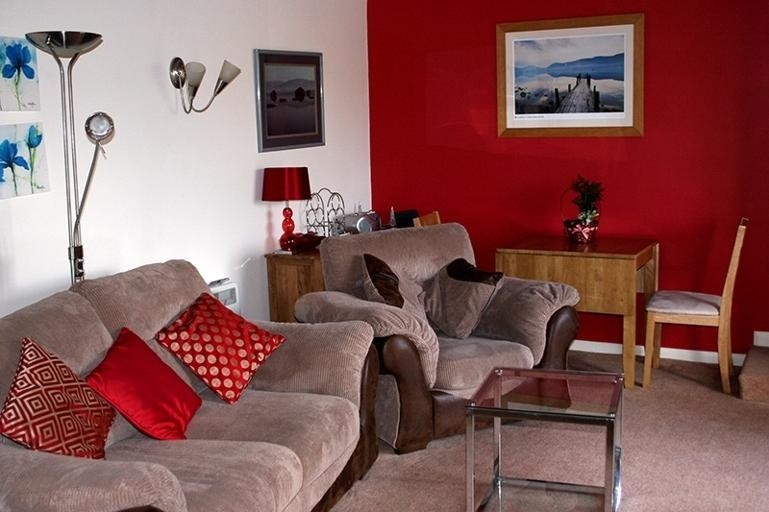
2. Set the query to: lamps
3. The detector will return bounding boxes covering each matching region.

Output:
[170,57,241,114]
[261,167,312,251]
[25,30,114,288]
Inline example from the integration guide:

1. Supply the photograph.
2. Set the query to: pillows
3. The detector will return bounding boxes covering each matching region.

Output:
[85,326,201,441]
[360,253,428,325]
[0,337,117,459]
[153,292,288,406]
[417,257,504,341]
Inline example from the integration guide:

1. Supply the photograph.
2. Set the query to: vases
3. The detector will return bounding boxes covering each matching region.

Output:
[564,219,598,244]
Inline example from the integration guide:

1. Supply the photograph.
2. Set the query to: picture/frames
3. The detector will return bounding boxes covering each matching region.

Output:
[495,12,646,140]
[252,49,326,154]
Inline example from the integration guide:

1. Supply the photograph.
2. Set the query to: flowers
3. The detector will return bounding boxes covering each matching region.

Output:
[560,174,603,224]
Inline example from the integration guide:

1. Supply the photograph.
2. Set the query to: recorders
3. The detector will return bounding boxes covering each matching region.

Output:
[334,212,381,234]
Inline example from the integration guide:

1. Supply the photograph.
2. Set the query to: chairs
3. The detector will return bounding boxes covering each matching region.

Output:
[293,220,581,455]
[641,217,750,395]
[413,210,440,229]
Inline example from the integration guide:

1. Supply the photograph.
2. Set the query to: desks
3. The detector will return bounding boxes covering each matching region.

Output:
[263,250,324,322]
[494,236,660,391]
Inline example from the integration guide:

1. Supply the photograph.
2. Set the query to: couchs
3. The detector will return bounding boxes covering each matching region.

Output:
[0,260,380,512]
